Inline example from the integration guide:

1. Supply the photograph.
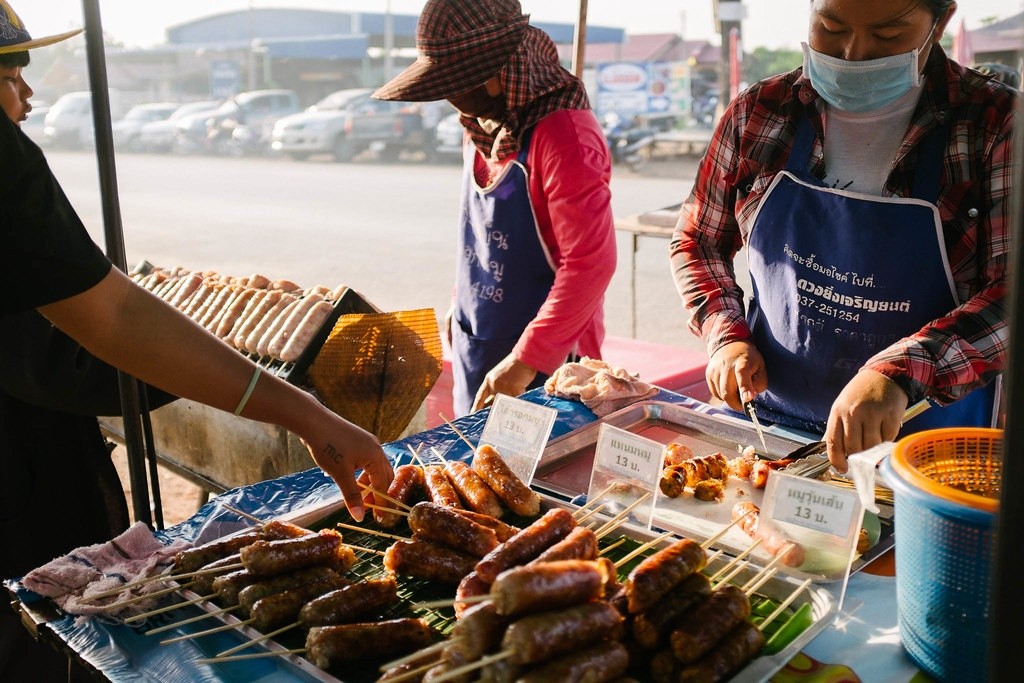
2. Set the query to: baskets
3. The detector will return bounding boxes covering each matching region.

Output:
[879,427,1007,683]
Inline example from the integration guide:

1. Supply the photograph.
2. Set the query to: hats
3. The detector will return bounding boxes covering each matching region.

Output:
[370,0,530,102]
[0,0,85,54]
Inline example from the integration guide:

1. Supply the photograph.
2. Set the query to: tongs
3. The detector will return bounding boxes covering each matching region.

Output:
[778,399,933,480]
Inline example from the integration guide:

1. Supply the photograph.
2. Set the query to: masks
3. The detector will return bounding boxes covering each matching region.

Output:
[800,17,939,114]
[448,82,494,118]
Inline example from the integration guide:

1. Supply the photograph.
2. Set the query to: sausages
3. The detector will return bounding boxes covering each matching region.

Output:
[131,266,385,365]
[176,443,871,683]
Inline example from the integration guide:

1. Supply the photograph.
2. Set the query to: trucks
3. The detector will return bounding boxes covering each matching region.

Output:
[343,104,434,162]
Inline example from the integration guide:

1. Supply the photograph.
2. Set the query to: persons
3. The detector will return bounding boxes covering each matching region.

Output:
[380,0,618,421]
[0,0,395,582]
[666,0,1024,475]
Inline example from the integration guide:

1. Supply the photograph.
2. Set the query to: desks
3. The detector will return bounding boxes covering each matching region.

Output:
[615,200,685,340]
[8,381,962,683]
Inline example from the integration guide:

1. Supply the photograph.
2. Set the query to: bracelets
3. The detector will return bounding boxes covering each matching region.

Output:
[231,362,264,416]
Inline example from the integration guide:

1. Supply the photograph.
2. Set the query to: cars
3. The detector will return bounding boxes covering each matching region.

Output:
[272,89,380,162]
[434,116,467,157]
[16,89,301,155]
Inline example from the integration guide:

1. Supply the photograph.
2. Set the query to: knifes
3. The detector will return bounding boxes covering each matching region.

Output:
[736,387,768,453]
